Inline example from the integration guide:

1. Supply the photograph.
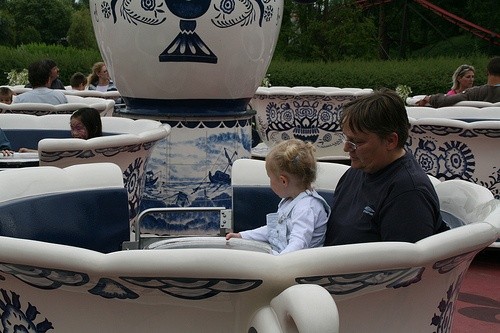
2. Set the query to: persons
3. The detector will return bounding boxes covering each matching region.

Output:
[446,65,475,96]
[19,106,102,154]
[0,128,14,156]
[322,88,442,248]
[0,60,68,106]
[415,56,500,109]
[86,62,126,108]
[70,72,88,91]
[226,139,332,255]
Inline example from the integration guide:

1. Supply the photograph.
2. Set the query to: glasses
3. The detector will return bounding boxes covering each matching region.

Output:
[343,137,379,149]
